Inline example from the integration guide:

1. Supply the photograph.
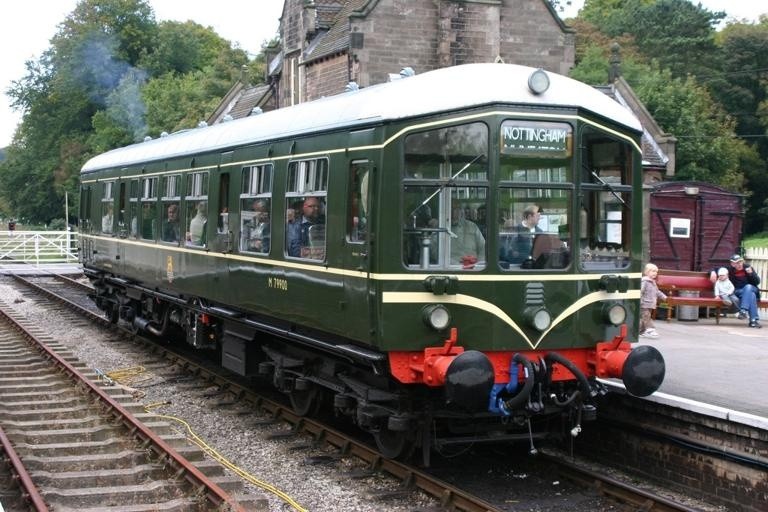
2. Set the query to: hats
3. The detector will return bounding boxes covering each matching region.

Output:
[729,255,743,262]
[717,267,729,276]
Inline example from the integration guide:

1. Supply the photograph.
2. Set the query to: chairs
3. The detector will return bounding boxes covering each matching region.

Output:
[142,209,580,260]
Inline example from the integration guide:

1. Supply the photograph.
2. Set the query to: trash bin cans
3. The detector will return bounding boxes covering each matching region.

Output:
[677,290,700,322]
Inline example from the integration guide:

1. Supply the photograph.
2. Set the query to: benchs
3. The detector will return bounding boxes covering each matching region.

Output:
[647,268,768,328]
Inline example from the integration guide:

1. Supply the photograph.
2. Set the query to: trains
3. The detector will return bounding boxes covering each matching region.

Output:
[74,60,676,466]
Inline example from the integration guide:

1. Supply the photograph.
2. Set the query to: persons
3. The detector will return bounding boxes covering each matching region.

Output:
[708,253,763,329]
[101,197,544,271]
[713,266,750,320]
[639,262,668,338]
[8,218,16,232]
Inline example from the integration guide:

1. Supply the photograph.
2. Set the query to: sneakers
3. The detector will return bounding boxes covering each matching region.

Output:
[638,328,660,339]
[738,312,747,319]
[749,321,762,327]
